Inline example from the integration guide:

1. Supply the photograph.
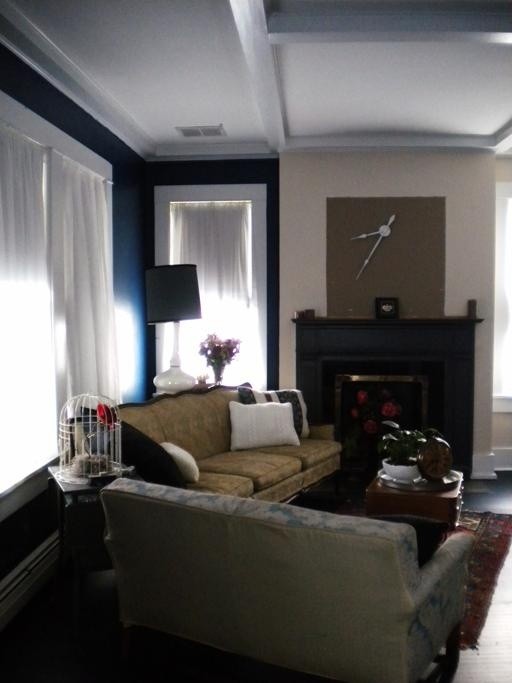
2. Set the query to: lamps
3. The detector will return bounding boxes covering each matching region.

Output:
[144,264,202,395]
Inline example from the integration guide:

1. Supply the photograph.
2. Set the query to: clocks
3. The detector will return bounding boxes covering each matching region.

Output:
[417,436,452,484]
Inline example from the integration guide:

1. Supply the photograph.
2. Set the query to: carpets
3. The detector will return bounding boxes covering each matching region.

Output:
[352,509,511,648]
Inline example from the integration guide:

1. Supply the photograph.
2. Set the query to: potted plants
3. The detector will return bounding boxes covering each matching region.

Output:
[382,421,420,484]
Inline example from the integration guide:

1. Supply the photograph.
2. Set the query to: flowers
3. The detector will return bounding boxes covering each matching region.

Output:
[200,334,241,365]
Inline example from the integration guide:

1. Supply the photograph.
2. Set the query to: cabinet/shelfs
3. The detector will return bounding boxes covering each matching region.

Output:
[290,318,484,479]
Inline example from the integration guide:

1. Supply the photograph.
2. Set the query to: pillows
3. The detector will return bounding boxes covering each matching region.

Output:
[237,385,311,439]
[228,400,301,451]
[160,441,199,481]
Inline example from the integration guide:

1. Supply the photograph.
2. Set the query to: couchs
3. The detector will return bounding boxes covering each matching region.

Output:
[100,477,476,683]
[109,383,343,508]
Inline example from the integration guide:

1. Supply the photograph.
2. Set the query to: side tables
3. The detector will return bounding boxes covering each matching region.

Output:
[46,467,146,592]
